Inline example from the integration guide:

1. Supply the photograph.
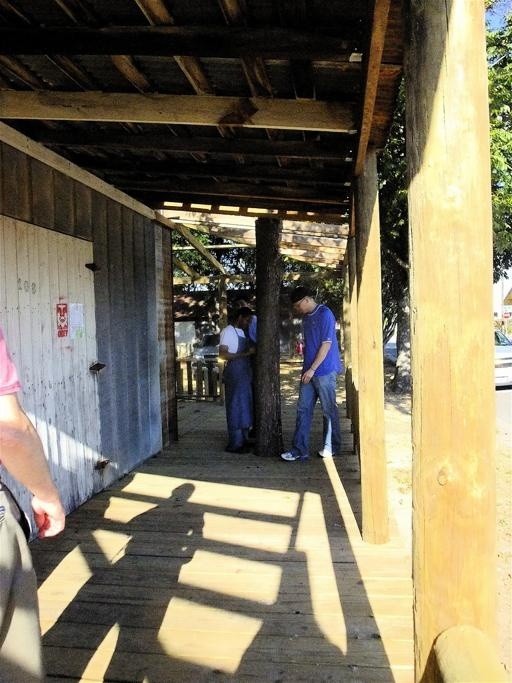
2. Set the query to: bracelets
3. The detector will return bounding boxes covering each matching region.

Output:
[310,367,316,371]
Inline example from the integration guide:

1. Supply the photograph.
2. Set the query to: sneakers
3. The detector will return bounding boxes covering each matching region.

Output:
[225,439,254,453]
[318,448,334,457]
[281,450,308,461]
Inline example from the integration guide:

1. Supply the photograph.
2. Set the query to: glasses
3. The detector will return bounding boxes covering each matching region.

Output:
[293,298,305,310]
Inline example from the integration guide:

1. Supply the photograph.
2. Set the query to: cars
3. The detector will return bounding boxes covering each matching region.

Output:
[189,333,220,377]
[494,328,512,388]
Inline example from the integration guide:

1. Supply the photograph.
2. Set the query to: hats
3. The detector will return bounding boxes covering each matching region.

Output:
[291,286,317,303]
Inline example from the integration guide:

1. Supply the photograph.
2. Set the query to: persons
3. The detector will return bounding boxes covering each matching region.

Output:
[218,305,255,454]
[204,295,256,363]
[1,322,67,682]
[279,286,344,462]
